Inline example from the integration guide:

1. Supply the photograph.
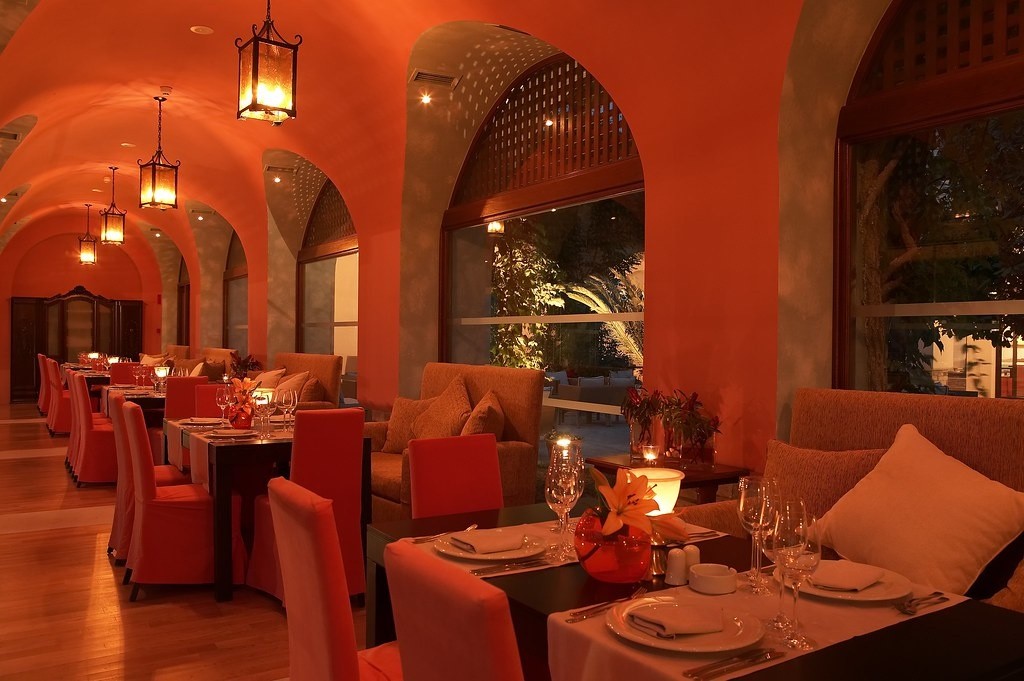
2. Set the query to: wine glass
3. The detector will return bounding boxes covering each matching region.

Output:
[544,442,585,562]
[736,475,821,651]
[215,384,298,439]
[78,351,132,376]
[133,365,189,397]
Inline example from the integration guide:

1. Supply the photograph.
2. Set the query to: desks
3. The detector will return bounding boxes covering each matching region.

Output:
[582,450,750,508]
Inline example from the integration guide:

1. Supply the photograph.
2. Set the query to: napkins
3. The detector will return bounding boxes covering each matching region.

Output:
[214,429,254,433]
[180,416,229,423]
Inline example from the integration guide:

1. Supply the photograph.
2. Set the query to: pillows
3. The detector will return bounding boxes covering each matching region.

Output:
[136,355,1024,595]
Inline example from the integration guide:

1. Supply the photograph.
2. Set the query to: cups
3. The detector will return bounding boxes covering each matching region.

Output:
[626,467,685,516]
[154,367,170,377]
[252,388,275,405]
[643,445,660,466]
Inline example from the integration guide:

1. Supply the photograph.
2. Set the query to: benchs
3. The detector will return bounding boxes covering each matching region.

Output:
[557,385,636,428]
[543,378,609,400]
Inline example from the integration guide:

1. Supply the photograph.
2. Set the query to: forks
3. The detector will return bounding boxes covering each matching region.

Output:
[893,591,949,615]
[412,523,478,544]
[565,586,647,624]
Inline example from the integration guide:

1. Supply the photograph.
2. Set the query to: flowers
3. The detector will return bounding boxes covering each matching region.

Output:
[622,386,721,465]
[587,467,661,536]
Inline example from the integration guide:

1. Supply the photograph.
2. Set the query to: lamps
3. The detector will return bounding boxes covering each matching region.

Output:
[137,95,181,210]
[625,468,684,576]
[78,204,98,265]
[98,167,127,245]
[486,221,504,238]
[235,0,303,126]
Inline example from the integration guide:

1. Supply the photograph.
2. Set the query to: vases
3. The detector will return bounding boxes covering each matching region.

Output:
[629,422,714,475]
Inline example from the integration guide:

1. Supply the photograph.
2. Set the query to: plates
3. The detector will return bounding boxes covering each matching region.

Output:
[179,418,225,424]
[773,559,912,601]
[204,430,258,438]
[434,533,546,560]
[257,416,295,421]
[606,596,765,652]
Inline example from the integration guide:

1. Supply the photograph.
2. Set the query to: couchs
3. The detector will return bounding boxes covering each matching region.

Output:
[165,345,189,360]
[274,354,343,404]
[364,362,542,544]
[201,347,237,382]
[666,390,1024,614]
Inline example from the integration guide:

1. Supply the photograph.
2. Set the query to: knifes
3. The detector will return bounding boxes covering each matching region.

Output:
[682,648,786,681]
[470,559,549,576]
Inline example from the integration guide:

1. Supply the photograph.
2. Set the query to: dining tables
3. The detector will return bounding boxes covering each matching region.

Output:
[61,361,89,387]
[394,519,1024,681]
[161,418,293,601]
[74,369,111,396]
[450,521,881,641]
[102,383,165,427]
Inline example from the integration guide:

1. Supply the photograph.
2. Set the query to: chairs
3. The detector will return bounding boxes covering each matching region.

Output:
[36,354,527,679]
